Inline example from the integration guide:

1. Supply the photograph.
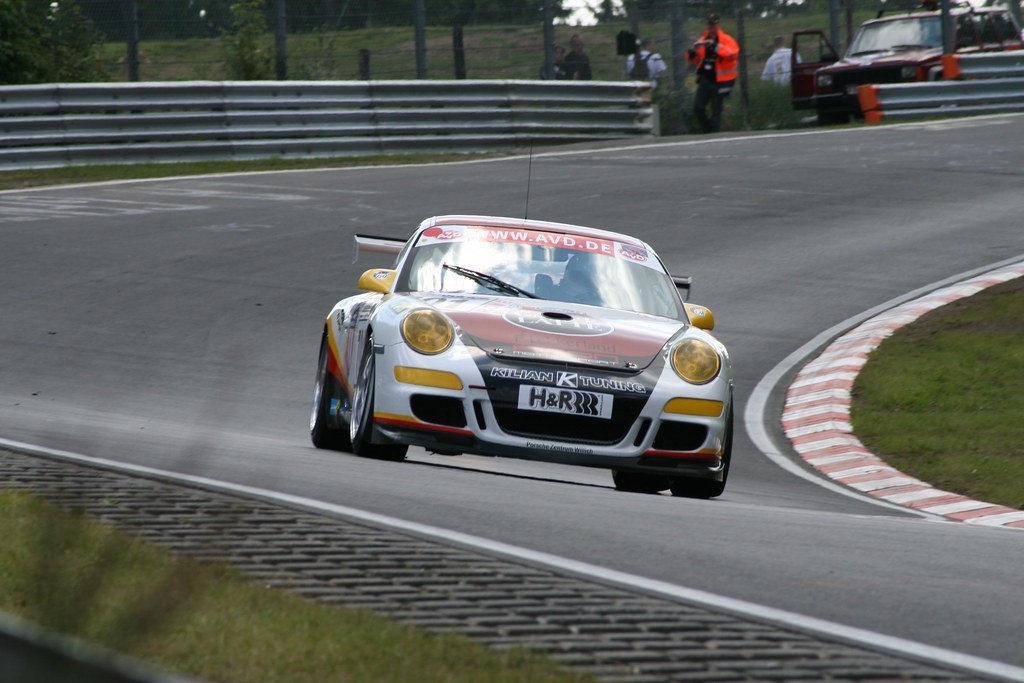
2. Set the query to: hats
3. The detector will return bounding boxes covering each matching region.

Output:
[706,14,719,23]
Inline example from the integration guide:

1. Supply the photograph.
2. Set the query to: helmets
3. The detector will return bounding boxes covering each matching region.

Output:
[561,254,612,308]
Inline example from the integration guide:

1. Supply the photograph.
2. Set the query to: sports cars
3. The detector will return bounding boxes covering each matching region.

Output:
[311,215,734,499]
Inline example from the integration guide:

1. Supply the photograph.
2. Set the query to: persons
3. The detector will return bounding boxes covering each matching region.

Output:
[761,36,801,85]
[627,38,667,85]
[684,14,739,133]
[554,34,593,80]
[563,254,610,305]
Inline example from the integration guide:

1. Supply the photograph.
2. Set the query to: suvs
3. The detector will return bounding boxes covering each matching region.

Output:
[791,6,1024,127]
